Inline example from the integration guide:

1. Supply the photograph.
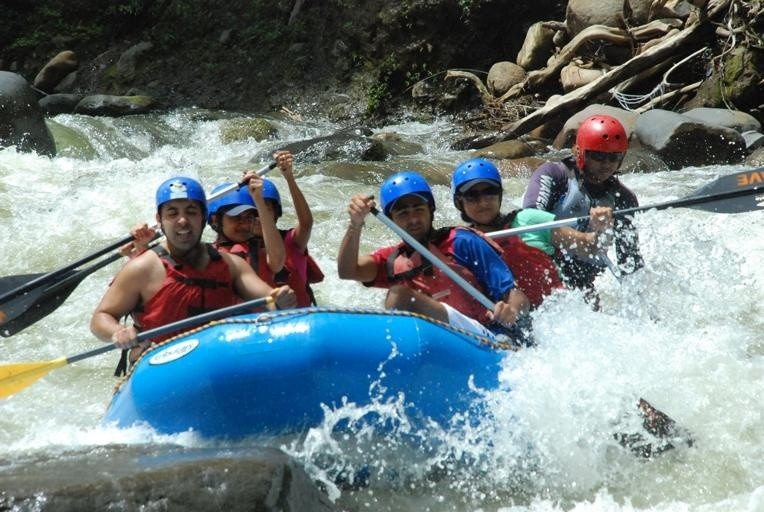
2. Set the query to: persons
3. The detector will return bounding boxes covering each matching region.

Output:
[118,182,286,314]
[240,151,316,308]
[450,157,615,314]
[520,115,646,312]
[337,169,537,347]
[90,177,300,362]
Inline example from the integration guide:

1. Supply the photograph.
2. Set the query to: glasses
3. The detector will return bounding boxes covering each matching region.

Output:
[460,187,504,197]
[588,151,623,162]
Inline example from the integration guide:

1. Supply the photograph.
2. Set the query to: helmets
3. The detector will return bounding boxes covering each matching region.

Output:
[156,176,282,224]
[452,159,502,213]
[380,172,435,220]
[575,114,628,171]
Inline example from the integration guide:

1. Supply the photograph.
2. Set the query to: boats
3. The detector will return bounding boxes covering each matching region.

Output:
[109,305,516,492]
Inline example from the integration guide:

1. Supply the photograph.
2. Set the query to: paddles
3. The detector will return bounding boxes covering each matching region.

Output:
[367,198,696,462]
[0,295,276,402]
[0,161,279,338]
[483,164,762,240]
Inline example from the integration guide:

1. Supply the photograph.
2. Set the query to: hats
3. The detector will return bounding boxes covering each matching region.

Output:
[387,192,430,213]
[457,178,500,195]
